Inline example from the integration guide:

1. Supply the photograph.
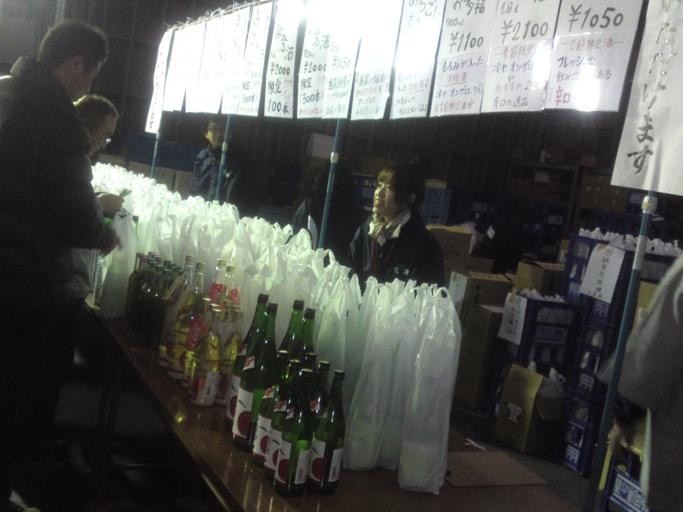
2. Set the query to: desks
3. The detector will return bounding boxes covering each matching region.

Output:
[102,314,580,511]
[71,263,128,430]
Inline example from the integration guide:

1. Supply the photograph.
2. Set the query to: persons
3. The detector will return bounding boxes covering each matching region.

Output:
[189,113,262,217]
[69,93,126,219]
[340,162,444,290]
[0,19,119,511]
[593,248,682,510]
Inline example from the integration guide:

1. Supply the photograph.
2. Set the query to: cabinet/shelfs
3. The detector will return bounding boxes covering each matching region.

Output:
[502,159,579,226]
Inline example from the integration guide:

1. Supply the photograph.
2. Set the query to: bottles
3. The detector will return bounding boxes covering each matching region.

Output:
[122,251,350,500]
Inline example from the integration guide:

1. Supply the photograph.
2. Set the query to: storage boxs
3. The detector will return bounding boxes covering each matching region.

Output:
[630,280,659,330]
[494,361,569,456]
[425,222,566,409]
